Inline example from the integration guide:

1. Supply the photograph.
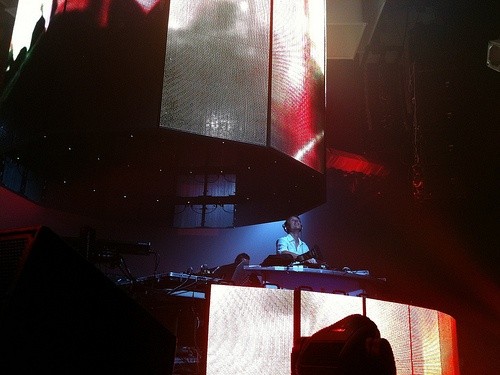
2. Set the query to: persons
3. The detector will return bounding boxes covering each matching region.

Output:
[274,214,317,267]
[213,252,262,286]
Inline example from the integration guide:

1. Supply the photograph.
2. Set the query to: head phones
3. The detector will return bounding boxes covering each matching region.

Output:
[284,216,304,232]
